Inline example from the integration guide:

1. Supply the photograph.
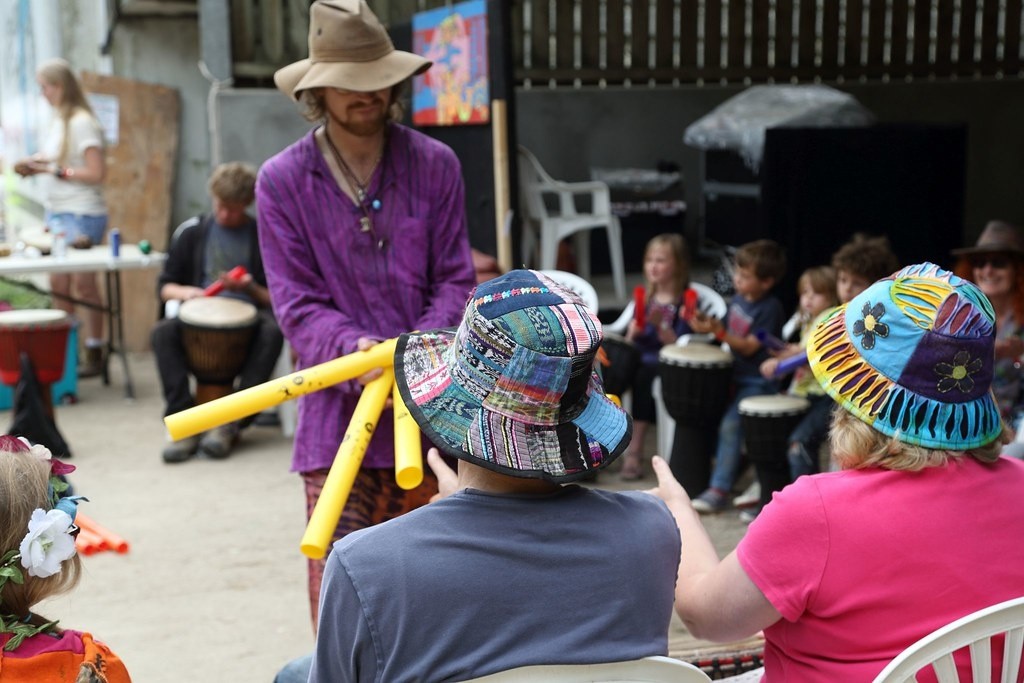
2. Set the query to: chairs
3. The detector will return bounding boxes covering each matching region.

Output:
[516,143,628,305]
[872,596,1024,683]
[457,654,712,683]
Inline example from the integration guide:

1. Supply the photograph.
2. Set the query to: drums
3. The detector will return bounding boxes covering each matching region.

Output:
[658,342,739,500]
[0,309,74,426]
[598,330,643,395]
[740,394,811,518]
[177,295,258,405]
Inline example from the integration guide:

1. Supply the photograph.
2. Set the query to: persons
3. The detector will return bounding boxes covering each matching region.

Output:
[13,59,108,377]
[598,221,1024,521]
[257,0,476,635]
[276,268,682,683]
[0,432,131,683]
[645,262,1024,683]
[149,164,285,463]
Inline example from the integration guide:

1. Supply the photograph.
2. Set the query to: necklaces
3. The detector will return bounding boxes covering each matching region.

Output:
[321,120,388,251]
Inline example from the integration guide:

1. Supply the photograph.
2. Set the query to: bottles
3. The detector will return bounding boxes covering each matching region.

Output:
[52,219,67,264]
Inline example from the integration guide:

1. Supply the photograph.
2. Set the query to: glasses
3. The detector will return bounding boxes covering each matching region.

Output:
[970,254,1009,268]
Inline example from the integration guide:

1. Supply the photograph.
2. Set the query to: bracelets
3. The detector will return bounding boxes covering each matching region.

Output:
[55,167,68,178]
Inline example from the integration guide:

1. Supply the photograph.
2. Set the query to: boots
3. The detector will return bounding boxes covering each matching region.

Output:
[76,345,102,376]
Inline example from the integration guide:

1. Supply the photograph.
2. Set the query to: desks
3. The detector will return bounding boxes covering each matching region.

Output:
[0,244,169,399]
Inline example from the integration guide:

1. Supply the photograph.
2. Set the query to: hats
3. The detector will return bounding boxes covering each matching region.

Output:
[955,219,1023,261]
[393,269,634,481]
[806,262,1006,450]
[272,0,432,103]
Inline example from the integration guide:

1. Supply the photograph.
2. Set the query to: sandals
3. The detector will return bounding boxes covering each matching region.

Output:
[622,451,643,481]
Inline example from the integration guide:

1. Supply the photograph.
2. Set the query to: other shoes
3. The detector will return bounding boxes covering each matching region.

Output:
[739,506,759,523]
[163,435,200,462]
[693,491,725,512]
[201,424,234,456]
[732,483,762,506]
